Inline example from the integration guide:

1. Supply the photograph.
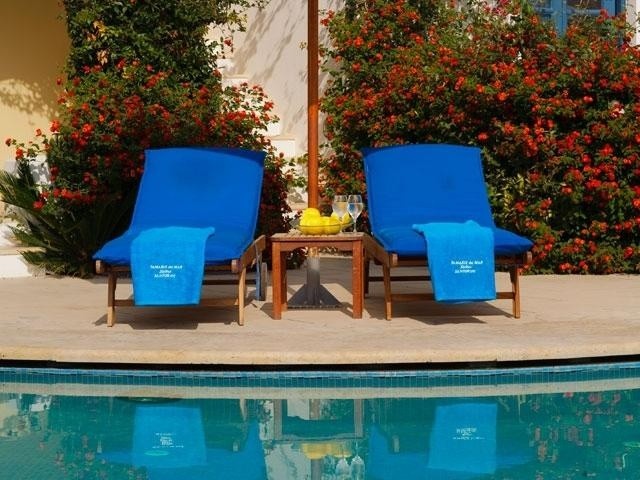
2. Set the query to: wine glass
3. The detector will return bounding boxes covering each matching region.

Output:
[336,439,365,480]
[331,195,364,235]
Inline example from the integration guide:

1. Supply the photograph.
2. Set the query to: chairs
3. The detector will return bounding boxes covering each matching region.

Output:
[92,147,267,328]
[362,145,536,322]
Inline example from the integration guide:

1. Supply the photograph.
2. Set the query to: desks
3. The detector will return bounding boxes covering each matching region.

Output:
[271,229,367,318]
[270,400,365,446]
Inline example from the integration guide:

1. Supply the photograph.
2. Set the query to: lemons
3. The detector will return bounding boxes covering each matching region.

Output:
[299,207,350,235]
[302,442,352,459]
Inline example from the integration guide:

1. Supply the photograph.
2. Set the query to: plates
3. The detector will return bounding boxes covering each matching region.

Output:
[288,217,354,235]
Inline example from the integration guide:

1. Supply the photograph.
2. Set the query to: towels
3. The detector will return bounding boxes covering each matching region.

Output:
[129,403,208,470]
[427,403,501,477]
[412,220,499,304]
[129,225,218,307]
